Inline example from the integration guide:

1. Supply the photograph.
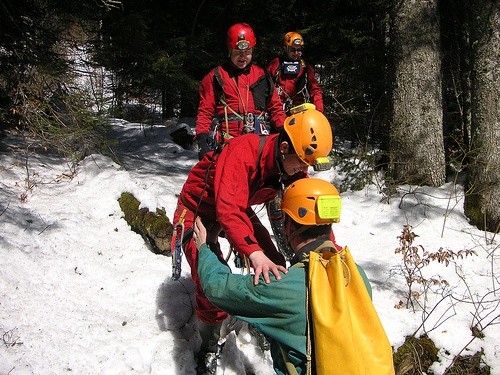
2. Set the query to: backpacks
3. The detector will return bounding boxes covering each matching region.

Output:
[276,246,396,375]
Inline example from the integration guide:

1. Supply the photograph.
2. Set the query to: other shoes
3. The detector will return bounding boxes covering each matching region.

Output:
[248,323,270,350]
[195,321,222,375]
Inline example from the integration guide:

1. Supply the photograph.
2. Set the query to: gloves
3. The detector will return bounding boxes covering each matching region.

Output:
[277,127,283,133]
[196,132,210,161]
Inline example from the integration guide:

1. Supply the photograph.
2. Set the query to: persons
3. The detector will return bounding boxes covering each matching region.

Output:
[195,21,288,161]
[192,177,372,374]
[173,102,333,375]
[266,31,324,114]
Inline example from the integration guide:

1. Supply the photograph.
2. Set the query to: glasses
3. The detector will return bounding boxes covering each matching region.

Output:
[288,48,301,52]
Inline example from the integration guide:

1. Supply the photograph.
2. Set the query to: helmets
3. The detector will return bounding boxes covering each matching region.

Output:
[227,23,256,50]
[283,32,305,48]
[283,103,333,166]
[280,178,341,224]
